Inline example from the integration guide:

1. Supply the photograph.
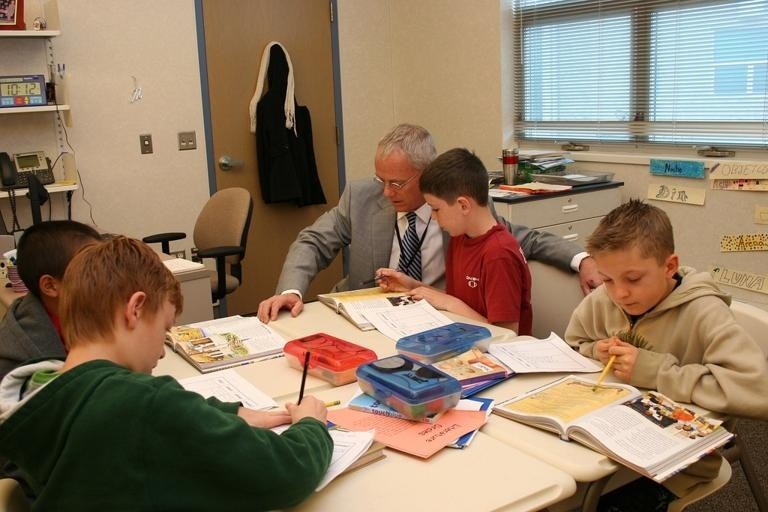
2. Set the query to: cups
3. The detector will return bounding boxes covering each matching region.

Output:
[373,174,420,191]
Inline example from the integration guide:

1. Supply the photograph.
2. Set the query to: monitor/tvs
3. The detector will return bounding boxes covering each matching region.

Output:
[492,373,737,486]
[163,258,204,274]
[317,287,420,332]
[500,181,573,195]
[165,314,285,374]
[313,346,517,476]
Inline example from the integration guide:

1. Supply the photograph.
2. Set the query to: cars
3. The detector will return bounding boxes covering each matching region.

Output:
[7,265,30,294]
[503,149,519,185]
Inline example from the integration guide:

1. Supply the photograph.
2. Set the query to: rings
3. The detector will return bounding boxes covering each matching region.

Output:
[592,355,616,391]
[360,268,402,285]
[298,352,310,405]
[324,400,340,406]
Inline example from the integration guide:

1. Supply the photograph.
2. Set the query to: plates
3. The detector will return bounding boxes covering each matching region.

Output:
[487,169,626,253]
[0,0,79,199]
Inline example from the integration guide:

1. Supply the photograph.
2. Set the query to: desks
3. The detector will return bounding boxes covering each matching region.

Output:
[0,244,215,327]
[150,286,746,512]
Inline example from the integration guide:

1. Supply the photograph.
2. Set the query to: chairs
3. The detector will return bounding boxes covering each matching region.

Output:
[140,185,255,317]
[662,298,768,512]
[527,256,586,342]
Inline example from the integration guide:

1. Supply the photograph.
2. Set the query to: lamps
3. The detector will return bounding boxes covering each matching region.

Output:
[527,256,586,342]
[662,298,768,512]
[140,185,255,317]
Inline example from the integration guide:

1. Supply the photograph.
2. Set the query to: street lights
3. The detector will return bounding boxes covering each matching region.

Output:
[256,44,328,207]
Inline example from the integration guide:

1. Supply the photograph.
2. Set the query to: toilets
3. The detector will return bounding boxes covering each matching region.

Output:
[0,151,55,191]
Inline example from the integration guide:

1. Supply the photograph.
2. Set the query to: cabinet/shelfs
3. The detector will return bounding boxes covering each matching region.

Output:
[487,169,626,253]
[0,0,79,199]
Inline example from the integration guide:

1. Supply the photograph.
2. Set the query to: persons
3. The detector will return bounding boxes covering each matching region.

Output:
[258,124,603,322]
[564,197,768,512]
[0,234,334,511]
[376,148,532,335]
[0,220,102,381]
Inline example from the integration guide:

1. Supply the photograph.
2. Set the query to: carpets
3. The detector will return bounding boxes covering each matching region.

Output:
[397,211,422,283]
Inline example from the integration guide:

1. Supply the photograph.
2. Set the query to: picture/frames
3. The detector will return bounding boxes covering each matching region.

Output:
[0,244,215,327]
